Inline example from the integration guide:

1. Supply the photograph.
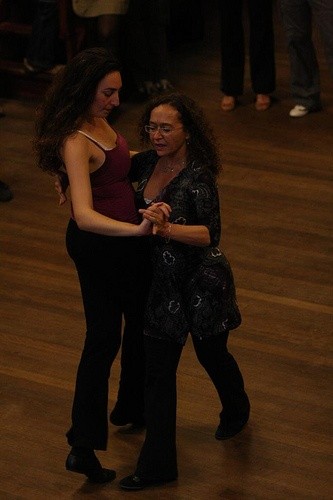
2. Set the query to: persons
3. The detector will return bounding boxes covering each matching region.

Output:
[32,48,172,483]
[54,94,250,490]
[280,0,333,117]
[24,0,126,72]
[219,0,276,111]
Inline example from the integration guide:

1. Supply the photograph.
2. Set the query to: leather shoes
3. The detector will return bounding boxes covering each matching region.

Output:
[119,473,177,491]
[110,407,142,426]
[215,401,250,441]
[65,450,117,483]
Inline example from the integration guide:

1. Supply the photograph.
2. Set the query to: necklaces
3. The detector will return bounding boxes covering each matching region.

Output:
[167,161,185,172]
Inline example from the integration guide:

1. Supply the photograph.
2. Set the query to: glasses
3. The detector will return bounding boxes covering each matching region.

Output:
[145,125,186,134]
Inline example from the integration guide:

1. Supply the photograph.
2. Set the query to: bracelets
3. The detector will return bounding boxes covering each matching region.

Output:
[165,223,172,243]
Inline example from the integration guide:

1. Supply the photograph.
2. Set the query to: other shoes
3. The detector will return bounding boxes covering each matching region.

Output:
[289,105,308,117]
[255,94,270,110]
[1,179,11,201]
[220,96,235,111]
